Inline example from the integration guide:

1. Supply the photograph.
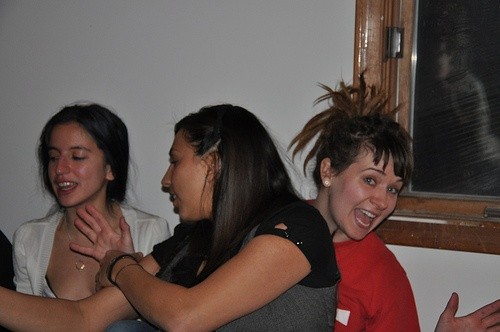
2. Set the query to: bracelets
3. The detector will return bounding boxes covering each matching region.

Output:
[114,263,141,286]
[107,253,137,285]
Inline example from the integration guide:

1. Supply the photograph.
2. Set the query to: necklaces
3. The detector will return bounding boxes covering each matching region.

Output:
[75,260,86,271]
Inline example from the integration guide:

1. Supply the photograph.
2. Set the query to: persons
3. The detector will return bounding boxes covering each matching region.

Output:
[420,36,500,197]
[0,103,339,332]
[286,68,500,332]
